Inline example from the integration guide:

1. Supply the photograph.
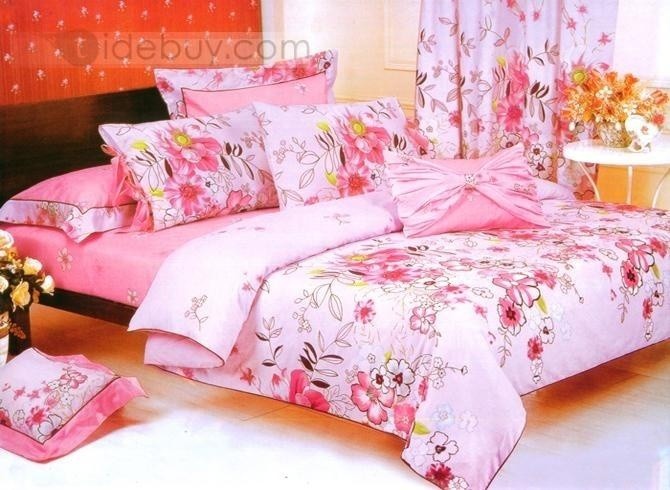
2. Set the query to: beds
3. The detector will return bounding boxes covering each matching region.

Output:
[1,86,670,490]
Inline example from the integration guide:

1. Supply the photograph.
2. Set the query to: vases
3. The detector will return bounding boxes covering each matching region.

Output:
[1,231,58,318]
[547,65,669,148]
[599,116,633,150]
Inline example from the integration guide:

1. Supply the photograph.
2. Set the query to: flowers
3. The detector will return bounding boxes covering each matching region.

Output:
[547,65,669,148]
[1,231,58,318]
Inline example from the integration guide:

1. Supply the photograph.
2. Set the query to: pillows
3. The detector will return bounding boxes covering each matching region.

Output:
[2,46,553,246]
[3,343,149,463]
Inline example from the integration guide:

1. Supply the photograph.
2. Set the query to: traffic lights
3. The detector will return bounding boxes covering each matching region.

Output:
[3,343,149,463]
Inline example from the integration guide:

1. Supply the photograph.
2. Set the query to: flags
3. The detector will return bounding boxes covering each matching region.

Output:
[599,116,633,150]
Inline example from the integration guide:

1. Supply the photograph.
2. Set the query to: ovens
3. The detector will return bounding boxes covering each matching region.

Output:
[1,86,670,490]
